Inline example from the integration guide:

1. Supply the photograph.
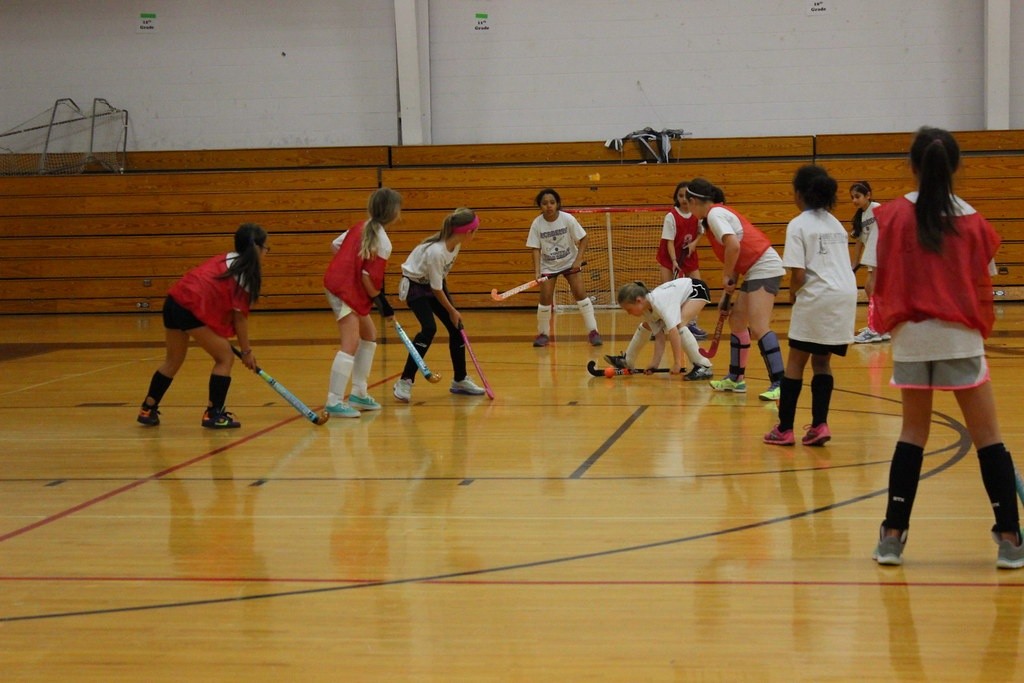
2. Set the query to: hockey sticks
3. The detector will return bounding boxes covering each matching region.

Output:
[393,321,443,384]
[458,321,495,400]
[672,250,688,280]
[1012,460,1024,505]
[698,279,734,358]
[587,360,687,377]
[853,262,861,272]
[490,261,588,301]
[229,345,330,426]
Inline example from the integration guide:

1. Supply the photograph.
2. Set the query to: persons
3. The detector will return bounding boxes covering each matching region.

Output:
[394,205,486,401]
[136,223,269,428]
[604,278,713,381]
[848,180,891,344]
[859,126,1024,570]
[655,182,704,284]
[762,165,858,445]
[687,177,786,401]
[525,189,602,347]
[323,187,403,417]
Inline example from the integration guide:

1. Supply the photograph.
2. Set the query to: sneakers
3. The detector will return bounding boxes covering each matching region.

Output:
[533,335,549,346]
[450,376,485,394]
[685,363,713,380]
[138,408,160,425]
[854,328,891,343]
[394,378,412,402]
[603,353,626,369]
[764,427,795,445]
[202,411,241,427]
[709,378,746,392]
[688,325,708,336]
[872,536,905,565]
[997,530,1024,568]
[802,423,831,445]
[589,330,602,345]
[324,403,361,417]
[759,387,780,400]
[349,394,380,410]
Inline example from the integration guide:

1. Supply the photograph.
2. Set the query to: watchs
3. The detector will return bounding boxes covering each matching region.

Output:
[241,349,252,355]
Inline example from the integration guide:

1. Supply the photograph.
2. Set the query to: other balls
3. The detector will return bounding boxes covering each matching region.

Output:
[604,367,614,378]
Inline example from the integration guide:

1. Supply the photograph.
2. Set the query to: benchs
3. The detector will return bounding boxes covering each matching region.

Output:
[0,127,1024,314]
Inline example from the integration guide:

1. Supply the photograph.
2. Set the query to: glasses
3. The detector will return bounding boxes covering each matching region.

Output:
[262,246,270,254]
